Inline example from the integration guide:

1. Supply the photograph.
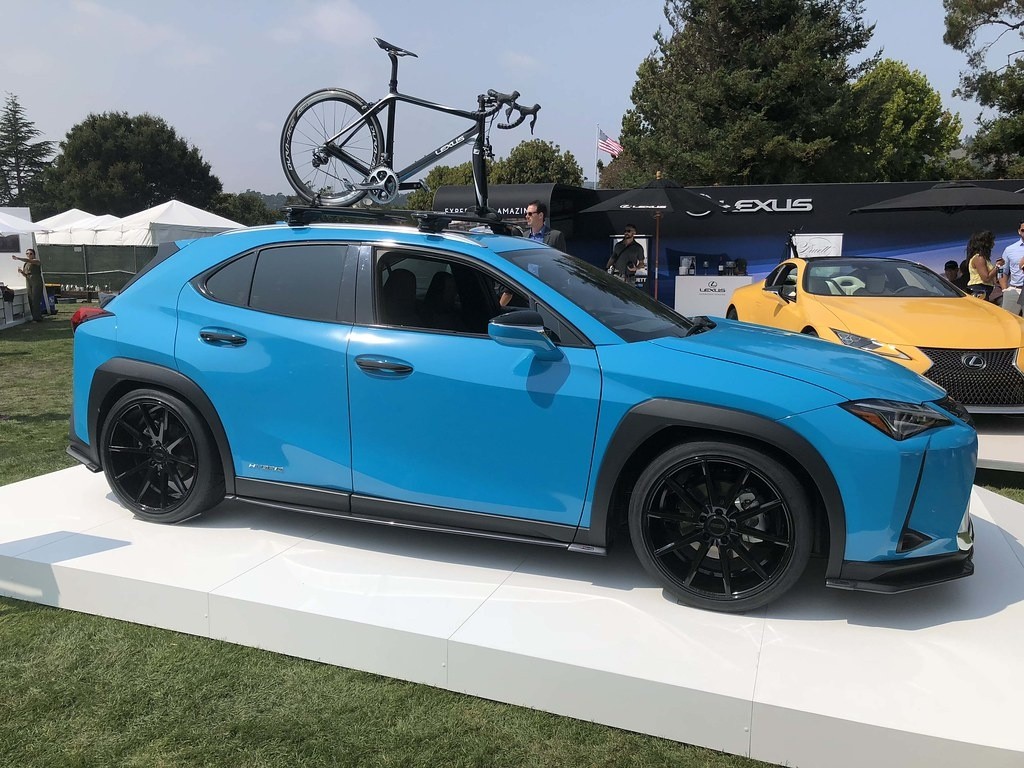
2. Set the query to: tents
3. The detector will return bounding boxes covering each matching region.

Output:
[0,199,250,315]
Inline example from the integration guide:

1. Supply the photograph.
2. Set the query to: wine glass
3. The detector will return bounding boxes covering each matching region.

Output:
[703,260,709,275]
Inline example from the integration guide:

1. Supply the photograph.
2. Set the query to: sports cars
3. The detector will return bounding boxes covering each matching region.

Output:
[725,256,1024,418]
[66,194,978,613]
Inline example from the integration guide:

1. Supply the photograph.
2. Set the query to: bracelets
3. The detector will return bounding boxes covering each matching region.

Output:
[993,266,999,271]
[996,263,1000,268]
[1021,264,1024,270]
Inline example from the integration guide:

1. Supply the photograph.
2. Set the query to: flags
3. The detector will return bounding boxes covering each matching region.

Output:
[598,128,623,158]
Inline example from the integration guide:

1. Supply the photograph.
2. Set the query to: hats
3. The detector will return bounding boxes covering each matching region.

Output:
[945,261,958,270]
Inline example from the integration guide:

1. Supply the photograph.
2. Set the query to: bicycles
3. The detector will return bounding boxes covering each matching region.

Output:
[280,37,542,216]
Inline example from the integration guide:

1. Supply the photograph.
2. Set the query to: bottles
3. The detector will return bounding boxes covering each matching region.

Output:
[689,260,695,275]
[997,265,1003,278]
[718,256,723,276]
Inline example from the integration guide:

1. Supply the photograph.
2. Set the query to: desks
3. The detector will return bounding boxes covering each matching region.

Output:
[675,275,754,318]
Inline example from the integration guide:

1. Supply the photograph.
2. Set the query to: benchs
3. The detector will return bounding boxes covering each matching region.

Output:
[782,275,864,297]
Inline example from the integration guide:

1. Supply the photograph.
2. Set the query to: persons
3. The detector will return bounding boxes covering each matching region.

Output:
[12,249,43,322]
[718,252,749,276]
[496,199,567,308]
[606,224,645,287]
[945,220,1024,319]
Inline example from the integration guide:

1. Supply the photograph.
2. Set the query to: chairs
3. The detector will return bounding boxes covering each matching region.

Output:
[421,272,465,330]
[381,268,420,325]
[853,271,893,297]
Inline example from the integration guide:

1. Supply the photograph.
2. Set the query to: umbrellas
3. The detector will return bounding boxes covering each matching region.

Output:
[847,178,1024,217]
[579,170,754,301]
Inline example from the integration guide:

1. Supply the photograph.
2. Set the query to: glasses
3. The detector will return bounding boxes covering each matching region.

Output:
[26,253,34,255]
[624,229,634,233]
[525,212,539,217]
[1019,229,1024,232]
